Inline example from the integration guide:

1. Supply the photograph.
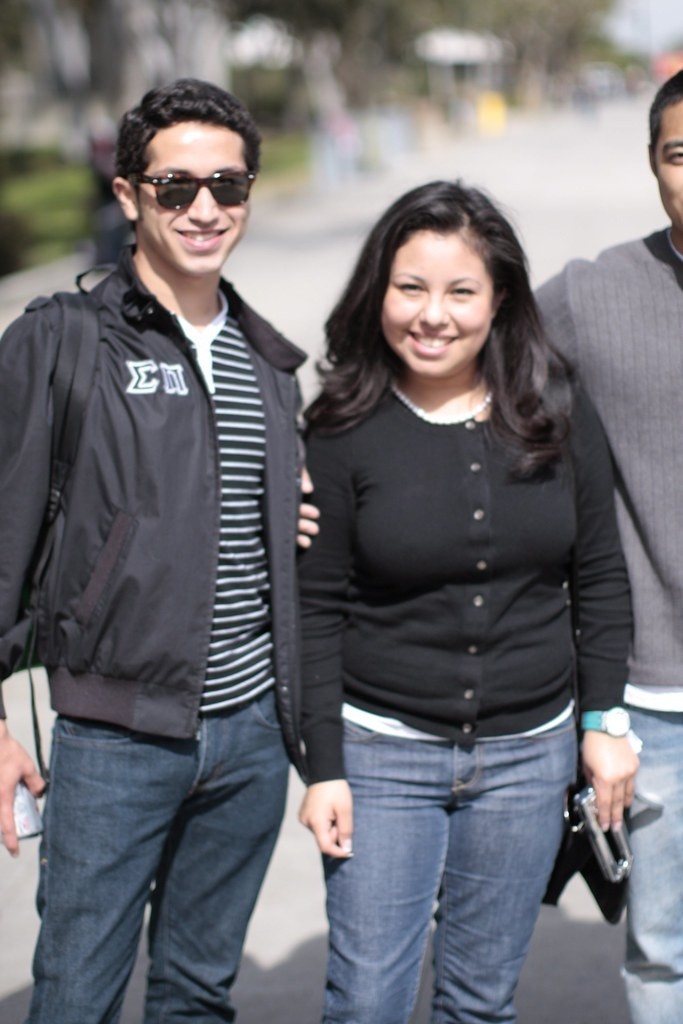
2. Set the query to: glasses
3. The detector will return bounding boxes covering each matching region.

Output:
[134,167,256,210]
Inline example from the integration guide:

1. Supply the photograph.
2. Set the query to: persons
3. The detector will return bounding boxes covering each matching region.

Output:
[0,80,309,1024]
[297,69,683,1024]
[297,181,638,1024]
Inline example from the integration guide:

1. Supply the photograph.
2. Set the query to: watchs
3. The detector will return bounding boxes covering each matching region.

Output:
[580,706,630,738]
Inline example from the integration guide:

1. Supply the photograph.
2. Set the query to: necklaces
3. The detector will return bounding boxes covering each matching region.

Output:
[392,383,494,426]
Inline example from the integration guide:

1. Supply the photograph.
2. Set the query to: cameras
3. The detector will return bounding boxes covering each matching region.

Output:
[12,781,43,840]
